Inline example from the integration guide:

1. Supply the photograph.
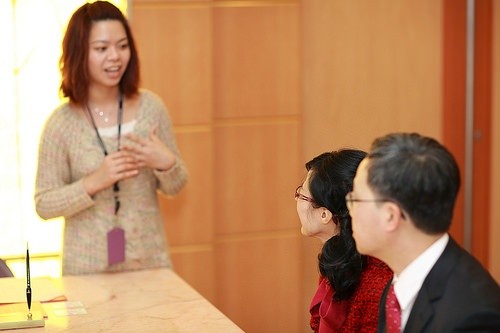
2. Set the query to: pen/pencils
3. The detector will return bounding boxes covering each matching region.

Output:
[26,249,31,310]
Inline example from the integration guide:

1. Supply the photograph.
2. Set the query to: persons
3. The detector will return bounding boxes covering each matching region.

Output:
[295,149,393,333]
[346,133,500,333]
[35,1,187,274]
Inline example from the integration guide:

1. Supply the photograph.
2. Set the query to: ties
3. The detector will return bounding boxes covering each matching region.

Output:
[385,284,401,333]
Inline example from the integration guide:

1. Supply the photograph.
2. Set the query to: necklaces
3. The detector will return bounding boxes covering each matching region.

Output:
[93,108,108,124]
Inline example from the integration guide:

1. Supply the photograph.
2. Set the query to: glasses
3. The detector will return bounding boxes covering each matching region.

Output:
[295,186,315,202]
[345,192,404,219]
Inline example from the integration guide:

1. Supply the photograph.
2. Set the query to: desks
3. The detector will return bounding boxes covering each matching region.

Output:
[0,269,247,333]
